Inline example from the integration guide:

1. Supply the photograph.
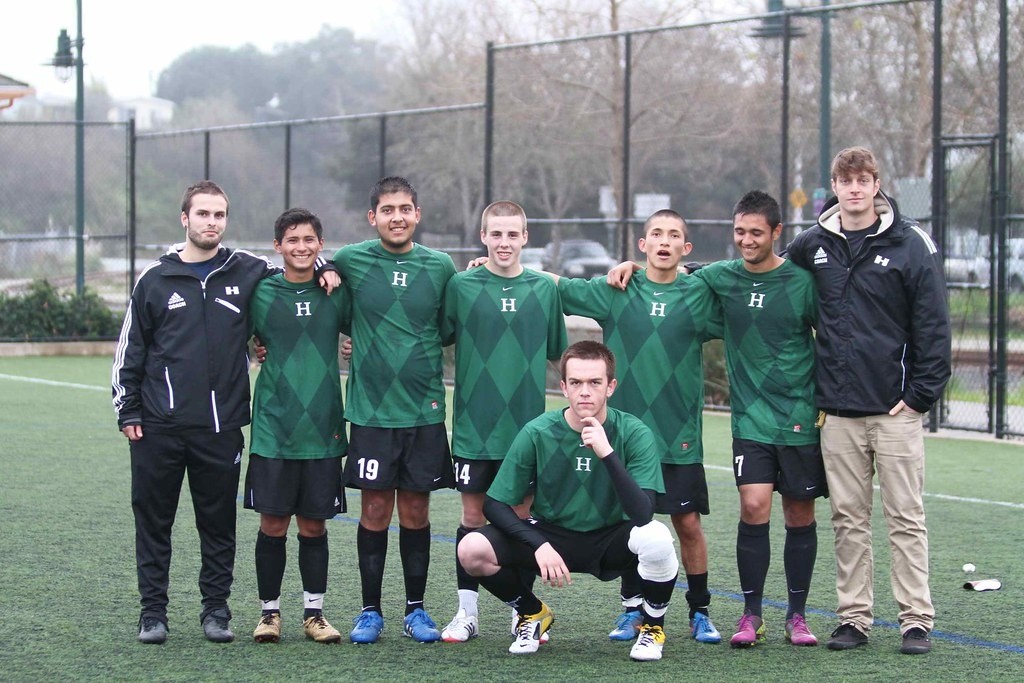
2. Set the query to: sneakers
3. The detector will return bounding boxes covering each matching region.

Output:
[403,608,441,643]
[689,612,721,643]
[900,627,932,655]
[630,624,666,661]
[137,618,167,643]
[508,600,555,654]
[511,609,549,644]
[302,614,341,642]
[826,623,868,651]
[203,609,234,642]
[784,612,818,646]
[252,613,282,644]
[441,608,479,643]
[729,608,766,647]
[350,611,383,645]
[608,611,644,641]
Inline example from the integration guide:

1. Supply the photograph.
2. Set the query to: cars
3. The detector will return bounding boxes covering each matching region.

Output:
[544,236,618,284]
[941,237,1024,290]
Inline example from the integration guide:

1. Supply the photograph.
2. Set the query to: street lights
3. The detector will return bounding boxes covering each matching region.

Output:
[752,0,833,215]
[42,0,85,304]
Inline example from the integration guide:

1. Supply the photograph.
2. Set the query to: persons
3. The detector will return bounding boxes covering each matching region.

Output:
[112,181,343,643]
[252,176,457,642]
[605,190,829,645]
[340,200,569,642]
[677,146,952,655]
[457,341,679,662]
[466,210,724,643]
[242,207,353,644]
